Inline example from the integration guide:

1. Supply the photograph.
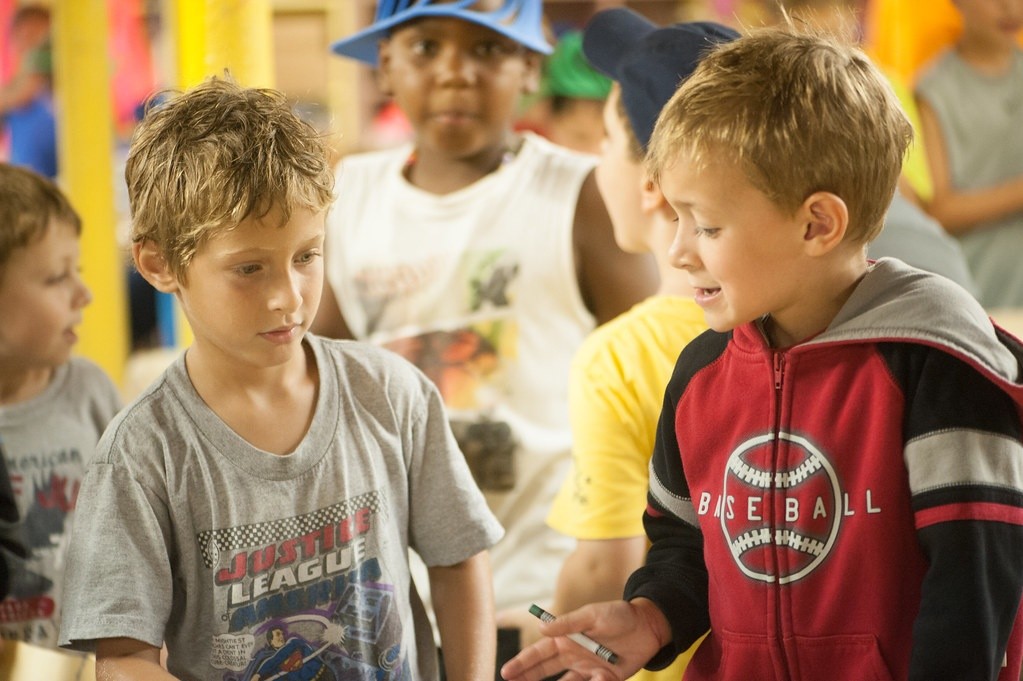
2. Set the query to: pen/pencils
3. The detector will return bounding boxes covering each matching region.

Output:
[528,604,618,665]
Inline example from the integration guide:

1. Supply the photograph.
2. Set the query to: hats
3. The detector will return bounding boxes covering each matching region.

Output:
[583,7,744,154]
[330,0,556,67]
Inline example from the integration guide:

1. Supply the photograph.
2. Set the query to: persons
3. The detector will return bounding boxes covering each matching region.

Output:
[295,0,661,681]
[0,1,60,178]
[0,156,123,651]
[537,31,620,159]
[56,76,511,681]
[520,7,750,654]
[498,27,1023,681]
[903,0,1022,342]
